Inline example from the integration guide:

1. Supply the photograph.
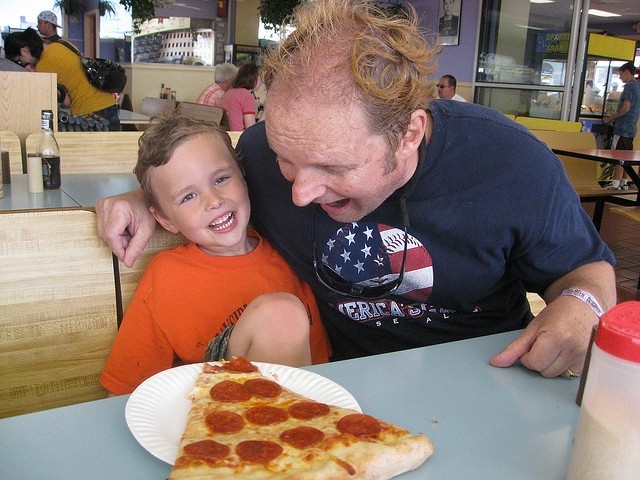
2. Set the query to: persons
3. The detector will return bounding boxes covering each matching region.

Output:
[36,11,63,42]
[4,26,127,132]
[602,63,640,190]
[439,0,458,36]
[95,0,617,379]
[435,74,467,102]
[195,63,239,106]
[98,116,334,398]
[221,64,259,131]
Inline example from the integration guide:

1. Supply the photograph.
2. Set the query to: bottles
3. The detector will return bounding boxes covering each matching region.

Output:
[166,88,171,101]
[35,108,63,192]
[159,83,166,100]
[171,91,176,101]
[562,304,640,480]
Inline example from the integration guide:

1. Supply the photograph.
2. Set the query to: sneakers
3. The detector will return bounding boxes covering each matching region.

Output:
[621,184,629,190]
[604,182,621,191]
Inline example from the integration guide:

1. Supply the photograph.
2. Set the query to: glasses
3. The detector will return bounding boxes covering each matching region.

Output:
[436,84,449,89]
[311,225,409,299]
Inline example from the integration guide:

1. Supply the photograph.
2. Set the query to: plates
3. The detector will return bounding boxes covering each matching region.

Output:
[125,354,368,468]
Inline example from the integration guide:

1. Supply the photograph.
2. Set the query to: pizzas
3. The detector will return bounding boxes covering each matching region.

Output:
[171,357,435,478]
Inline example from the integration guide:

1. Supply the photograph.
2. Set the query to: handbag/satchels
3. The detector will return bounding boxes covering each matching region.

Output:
[78,52,127,93]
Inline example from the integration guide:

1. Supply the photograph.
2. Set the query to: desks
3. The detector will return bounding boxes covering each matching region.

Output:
[551,148,640,206]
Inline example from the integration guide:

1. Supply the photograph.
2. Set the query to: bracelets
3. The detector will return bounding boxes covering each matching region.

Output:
[561,288,604,318]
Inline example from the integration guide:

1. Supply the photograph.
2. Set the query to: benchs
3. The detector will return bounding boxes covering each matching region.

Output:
[528,129,638,236]
[139,97,177,129]
[25,130,244,173]
[177,101,224,125]
[118,223,547,317]
[1,131,23,174]
[1,209,119,421]
[504,114,515,121]
[516,117,583,132]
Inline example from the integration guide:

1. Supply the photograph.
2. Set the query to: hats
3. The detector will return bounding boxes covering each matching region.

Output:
[37,11,63,29]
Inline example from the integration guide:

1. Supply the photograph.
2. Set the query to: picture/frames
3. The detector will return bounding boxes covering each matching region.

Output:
[437,0,462,46]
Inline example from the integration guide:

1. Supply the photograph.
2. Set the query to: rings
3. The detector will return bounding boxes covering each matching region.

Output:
[566,369,580,379]
